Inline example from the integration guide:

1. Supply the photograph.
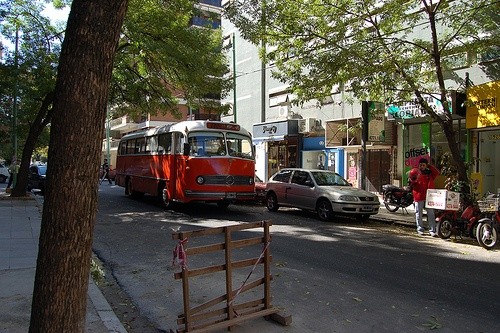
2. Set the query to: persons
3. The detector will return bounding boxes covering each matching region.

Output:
[223,141,233,154]
[409,158,440,237]
[7,161,16,188]
[100,159,112,184]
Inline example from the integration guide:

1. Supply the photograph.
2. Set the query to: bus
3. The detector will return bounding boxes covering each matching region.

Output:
[115,119,257,210]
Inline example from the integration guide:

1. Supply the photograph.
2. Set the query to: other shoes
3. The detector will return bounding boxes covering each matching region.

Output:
[418,231,424,235]
[430,232,436,236]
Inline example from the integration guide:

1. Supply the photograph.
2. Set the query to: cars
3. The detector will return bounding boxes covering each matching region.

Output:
[0,159,48,191]
[233,169,266,205]
[264,168,381,223]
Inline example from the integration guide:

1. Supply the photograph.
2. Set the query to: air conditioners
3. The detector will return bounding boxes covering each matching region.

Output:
[297,118,316,134]
[279,106,291,117]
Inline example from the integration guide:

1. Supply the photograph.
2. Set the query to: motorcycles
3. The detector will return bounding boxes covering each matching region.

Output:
[382,178,426,216]
[434,180,500,251]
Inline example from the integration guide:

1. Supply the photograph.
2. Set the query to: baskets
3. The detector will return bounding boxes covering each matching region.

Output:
[477,198,499,213]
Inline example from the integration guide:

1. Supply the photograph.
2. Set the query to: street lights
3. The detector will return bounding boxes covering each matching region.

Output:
[222,32,238,152]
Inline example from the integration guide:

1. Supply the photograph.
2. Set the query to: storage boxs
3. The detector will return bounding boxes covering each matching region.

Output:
[477,198,500,212]
[425,189,460,210]
